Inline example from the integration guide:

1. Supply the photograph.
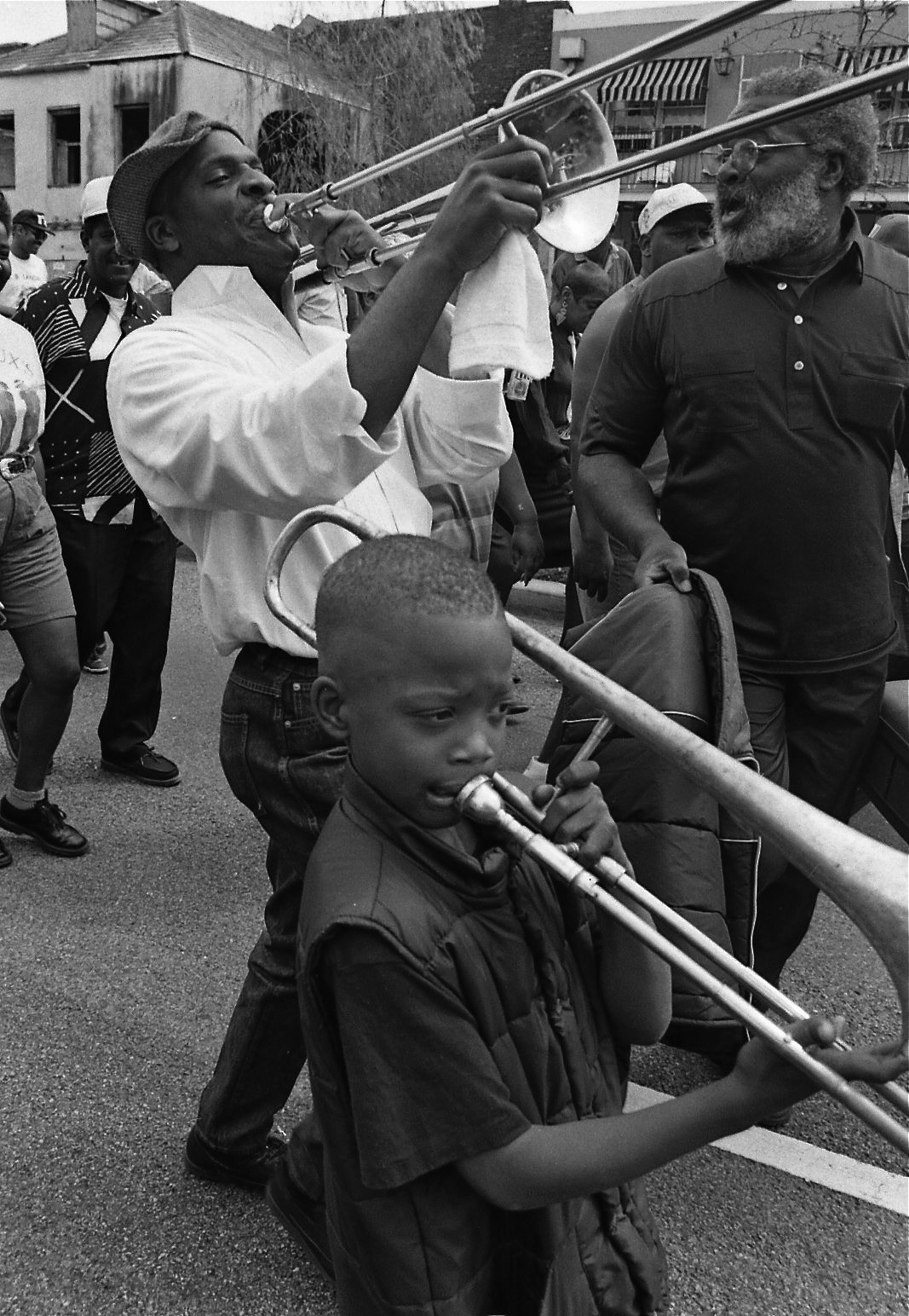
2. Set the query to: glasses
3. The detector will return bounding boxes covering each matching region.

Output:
[700,142,835,177]
[24,227,49,241]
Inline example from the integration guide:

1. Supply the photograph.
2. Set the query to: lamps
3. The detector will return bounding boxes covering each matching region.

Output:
[713,45,735,76]
[802,40,825,67]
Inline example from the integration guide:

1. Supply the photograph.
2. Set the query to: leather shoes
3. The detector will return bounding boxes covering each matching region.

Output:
[0,789,89,858]
[0,839,13,867]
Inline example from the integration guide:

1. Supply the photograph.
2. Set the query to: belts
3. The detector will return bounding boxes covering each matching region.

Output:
[0,457,29,479]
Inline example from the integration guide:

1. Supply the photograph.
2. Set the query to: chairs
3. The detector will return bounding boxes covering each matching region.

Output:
[633,159,677,189]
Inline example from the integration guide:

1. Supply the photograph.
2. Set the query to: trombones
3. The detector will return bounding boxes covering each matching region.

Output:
[263,504,908,1158]
[261,0,909,296]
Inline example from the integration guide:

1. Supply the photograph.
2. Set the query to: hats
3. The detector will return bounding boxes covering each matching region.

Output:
[108,109,247,276]
[13,209,56,238]
[635,183,715,235]
[80,177,117,223]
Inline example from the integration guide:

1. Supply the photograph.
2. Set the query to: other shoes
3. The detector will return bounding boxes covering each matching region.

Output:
[185,1126,293,1198]
[264,1173,333,1290]
[0,706,55,776]
[84,654,108,673]
[100,743,182,786]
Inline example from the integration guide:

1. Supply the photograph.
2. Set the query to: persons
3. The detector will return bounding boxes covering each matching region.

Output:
[294,534,908,1316]
[0,65,908,1293]
